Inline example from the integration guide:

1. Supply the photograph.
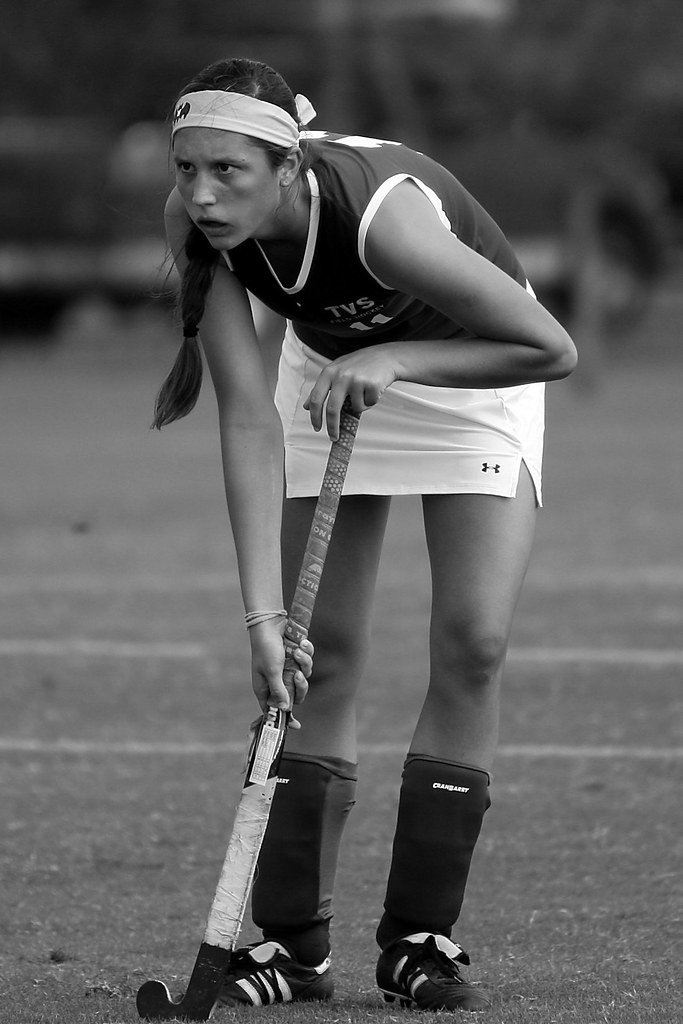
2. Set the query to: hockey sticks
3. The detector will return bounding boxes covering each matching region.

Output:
[134,402,364,1024]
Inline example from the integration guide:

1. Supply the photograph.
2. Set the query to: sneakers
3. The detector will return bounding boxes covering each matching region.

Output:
[376,935,490,1015]
[217,940,333,1009]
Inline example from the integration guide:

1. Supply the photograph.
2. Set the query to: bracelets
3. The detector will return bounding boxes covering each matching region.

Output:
[244,609,287,629]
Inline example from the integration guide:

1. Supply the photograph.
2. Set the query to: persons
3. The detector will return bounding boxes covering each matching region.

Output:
[163,58,578,1016]
